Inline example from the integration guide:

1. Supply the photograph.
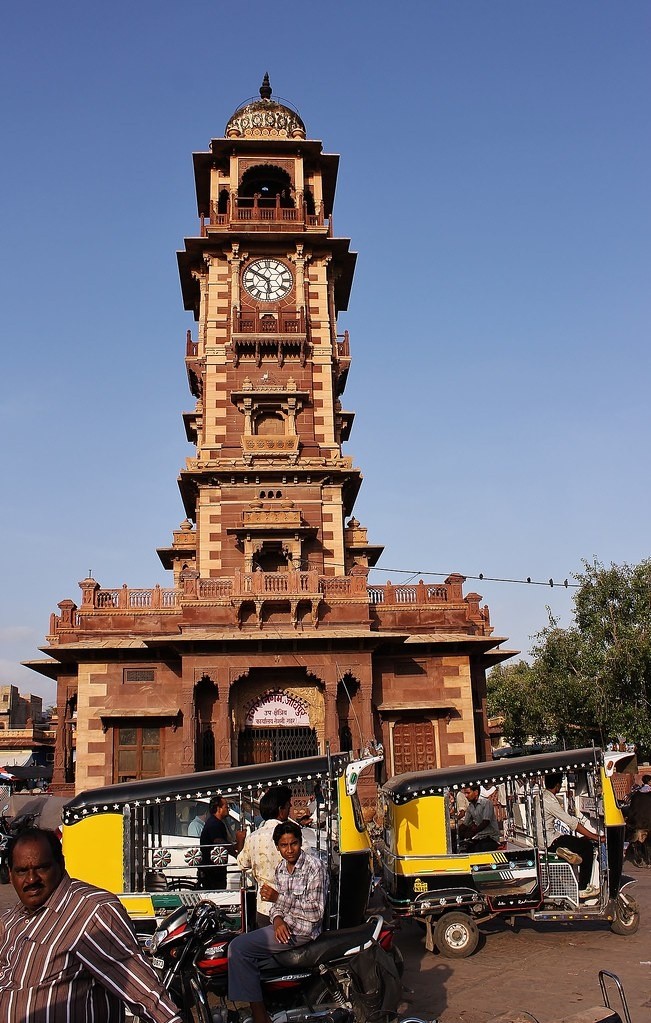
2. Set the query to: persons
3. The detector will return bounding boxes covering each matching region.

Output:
[187,772,651,1023]
[0,827,182,1023]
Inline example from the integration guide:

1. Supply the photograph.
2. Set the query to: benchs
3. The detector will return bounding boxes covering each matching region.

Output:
[506,838,566,864]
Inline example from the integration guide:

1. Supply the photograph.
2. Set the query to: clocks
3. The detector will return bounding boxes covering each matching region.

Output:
[243,258,292,302]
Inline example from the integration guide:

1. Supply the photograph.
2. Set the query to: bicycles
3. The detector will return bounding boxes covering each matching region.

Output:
[627,827,651,868]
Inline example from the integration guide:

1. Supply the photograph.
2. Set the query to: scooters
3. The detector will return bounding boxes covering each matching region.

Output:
[0,805,42,885]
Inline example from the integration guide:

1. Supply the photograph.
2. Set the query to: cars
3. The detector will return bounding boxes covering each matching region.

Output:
[56,793,331,892]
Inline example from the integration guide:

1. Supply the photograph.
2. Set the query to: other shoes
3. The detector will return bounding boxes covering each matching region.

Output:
[637,862,643,867]
[647,864,651,869]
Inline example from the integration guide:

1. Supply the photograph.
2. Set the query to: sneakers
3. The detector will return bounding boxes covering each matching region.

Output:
[578,886,600,898]
[556,848,583,866]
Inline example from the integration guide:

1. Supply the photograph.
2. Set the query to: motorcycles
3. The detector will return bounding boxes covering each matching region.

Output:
[135,896,415,1023]
[373,742,642,961]
[361,967,632,1023]
[55,737,386,955]
